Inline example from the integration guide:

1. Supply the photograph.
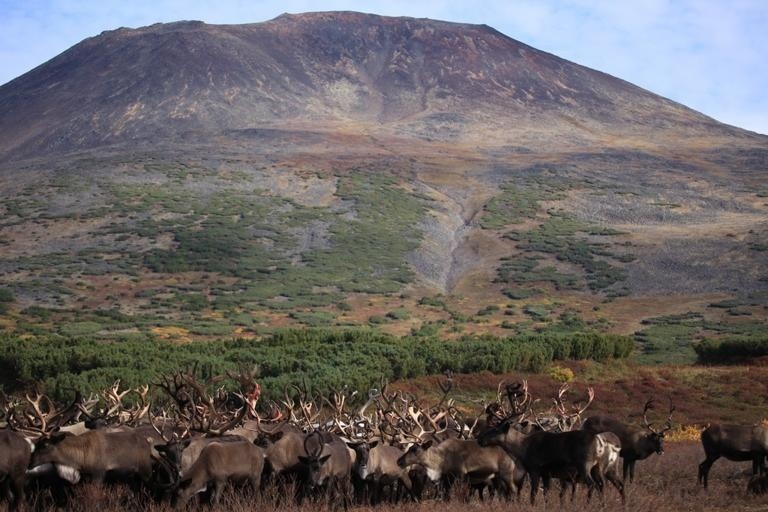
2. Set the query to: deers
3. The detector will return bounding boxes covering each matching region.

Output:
[581,392,679,487]
[695,421,768,490]
[0,360,628,512]
